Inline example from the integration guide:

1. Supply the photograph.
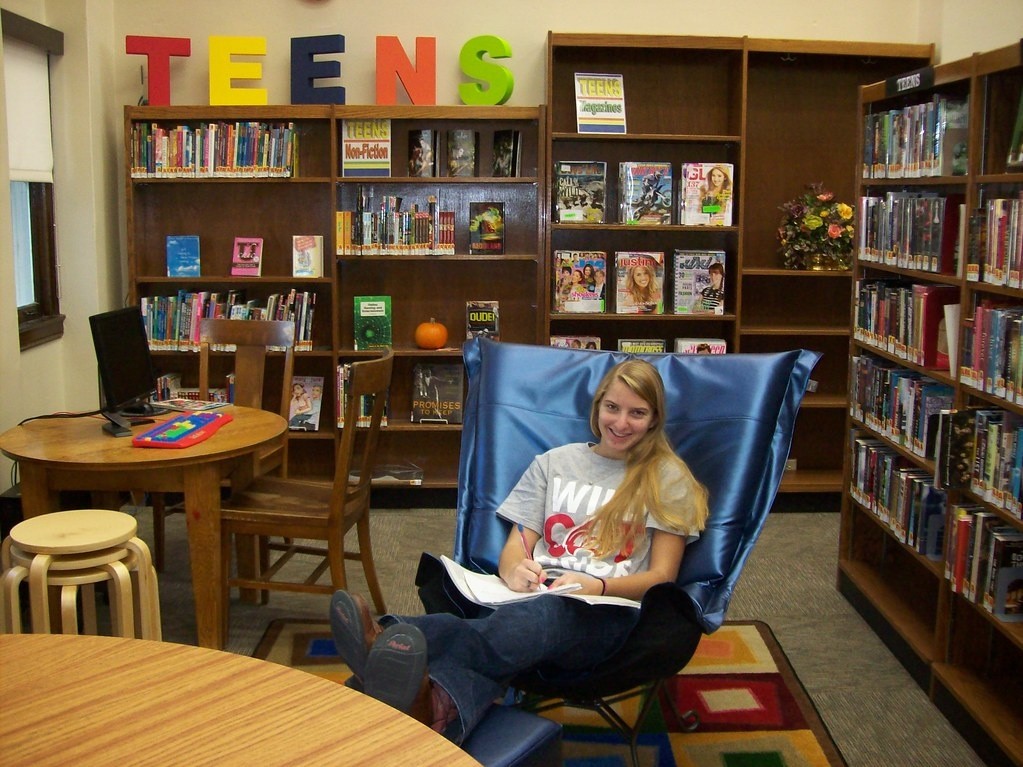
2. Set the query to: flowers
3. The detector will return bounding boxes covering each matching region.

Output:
[776,182,855,271]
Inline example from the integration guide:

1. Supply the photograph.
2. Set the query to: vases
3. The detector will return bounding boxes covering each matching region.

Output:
[802,253,853,271]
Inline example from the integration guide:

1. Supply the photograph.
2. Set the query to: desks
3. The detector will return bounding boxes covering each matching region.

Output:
[1,633,483,766]
[0,406,288,650]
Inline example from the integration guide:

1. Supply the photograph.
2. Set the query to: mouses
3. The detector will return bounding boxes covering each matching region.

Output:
[123,402,154,415]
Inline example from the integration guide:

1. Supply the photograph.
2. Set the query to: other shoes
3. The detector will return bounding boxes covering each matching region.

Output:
[361,625,456,735]
[330,588,385,690]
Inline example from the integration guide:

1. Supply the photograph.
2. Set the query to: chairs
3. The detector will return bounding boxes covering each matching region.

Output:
[454,339,824,767]
[217,345,394,650]
[152,318,296,601]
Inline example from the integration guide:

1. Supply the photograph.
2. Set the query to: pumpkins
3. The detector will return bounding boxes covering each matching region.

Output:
[414,318,447,349]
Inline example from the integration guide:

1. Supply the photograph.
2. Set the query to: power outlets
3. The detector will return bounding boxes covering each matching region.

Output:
[785,459,797,470]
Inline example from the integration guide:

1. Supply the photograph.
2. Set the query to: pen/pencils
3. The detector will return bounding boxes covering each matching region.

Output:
[517,523,543,591]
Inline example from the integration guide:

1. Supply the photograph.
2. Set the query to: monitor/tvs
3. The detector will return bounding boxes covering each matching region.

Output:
[88,306,156,437]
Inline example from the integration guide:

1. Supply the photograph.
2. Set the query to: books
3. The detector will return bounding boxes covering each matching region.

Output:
[554,161,607,224]
[166,236,200,277]
[574,73,627,134]
[230,238,263,277]
[850,90,1023,619]
[131,123,299,178]
[293,235,324,278]
[140,290,315,353]
[149,374,234,411]
[553,250,606,314]
[337,296,499,426]
[289,376,324,431]
[336,119,522,255]
[550,336,602,350]
[614,160,733,354]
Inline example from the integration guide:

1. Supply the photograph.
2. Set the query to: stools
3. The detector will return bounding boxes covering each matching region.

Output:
[346,673,562,767]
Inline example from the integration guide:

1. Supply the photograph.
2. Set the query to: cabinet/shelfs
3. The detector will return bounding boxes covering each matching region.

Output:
[546,31,940,512]
[124,104,547,510]
[831,38,1022,767]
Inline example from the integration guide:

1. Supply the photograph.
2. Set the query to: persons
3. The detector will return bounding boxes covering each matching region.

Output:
[572,339,596,349]
[700,165,733,225]
[692,263,724,314]
[621,264,661,314]
[558,264,605,300]
[329,359,708,747]
[641,171,662,200]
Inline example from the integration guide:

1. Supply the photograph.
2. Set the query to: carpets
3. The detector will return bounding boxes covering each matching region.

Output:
[250,618,849,767]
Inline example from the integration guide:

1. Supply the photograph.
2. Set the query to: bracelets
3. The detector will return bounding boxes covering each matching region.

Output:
[598,578,608,596]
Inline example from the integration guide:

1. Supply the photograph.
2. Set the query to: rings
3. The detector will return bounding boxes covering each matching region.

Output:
[527,580,531,588]
